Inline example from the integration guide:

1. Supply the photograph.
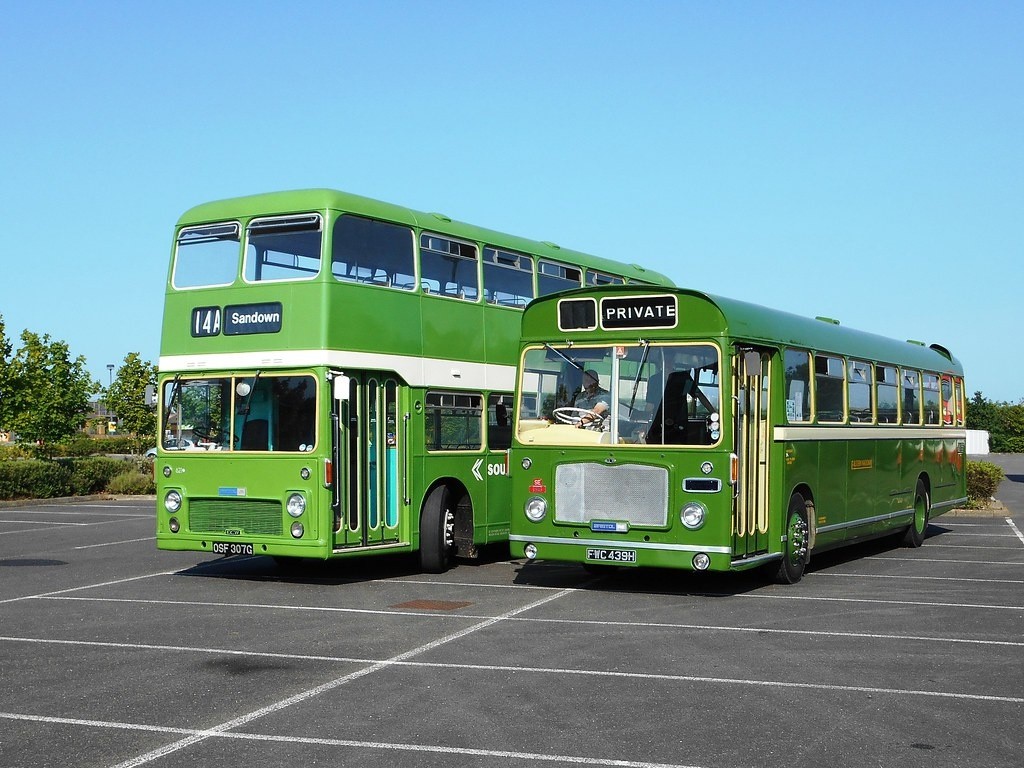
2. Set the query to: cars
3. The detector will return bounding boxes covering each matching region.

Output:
[194,441,228,451]
[143,437,207,460]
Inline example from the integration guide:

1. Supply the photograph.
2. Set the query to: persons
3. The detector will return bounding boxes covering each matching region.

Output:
[645,349,716,444]
[569,370,611,428]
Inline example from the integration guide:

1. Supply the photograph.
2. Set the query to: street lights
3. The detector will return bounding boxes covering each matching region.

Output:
[107,364,115,436]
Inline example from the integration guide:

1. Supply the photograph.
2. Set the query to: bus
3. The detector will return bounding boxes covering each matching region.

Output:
[148,188,679,575]
[508,284,968,585]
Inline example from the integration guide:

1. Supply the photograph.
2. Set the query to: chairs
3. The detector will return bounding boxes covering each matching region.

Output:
[401,281,430,293]
[436,287,464,300]
[468,295,498,305]
[803,407,939,424]
[361,275,390,287]
[240,419,268,450]
[497,298,527,309]
